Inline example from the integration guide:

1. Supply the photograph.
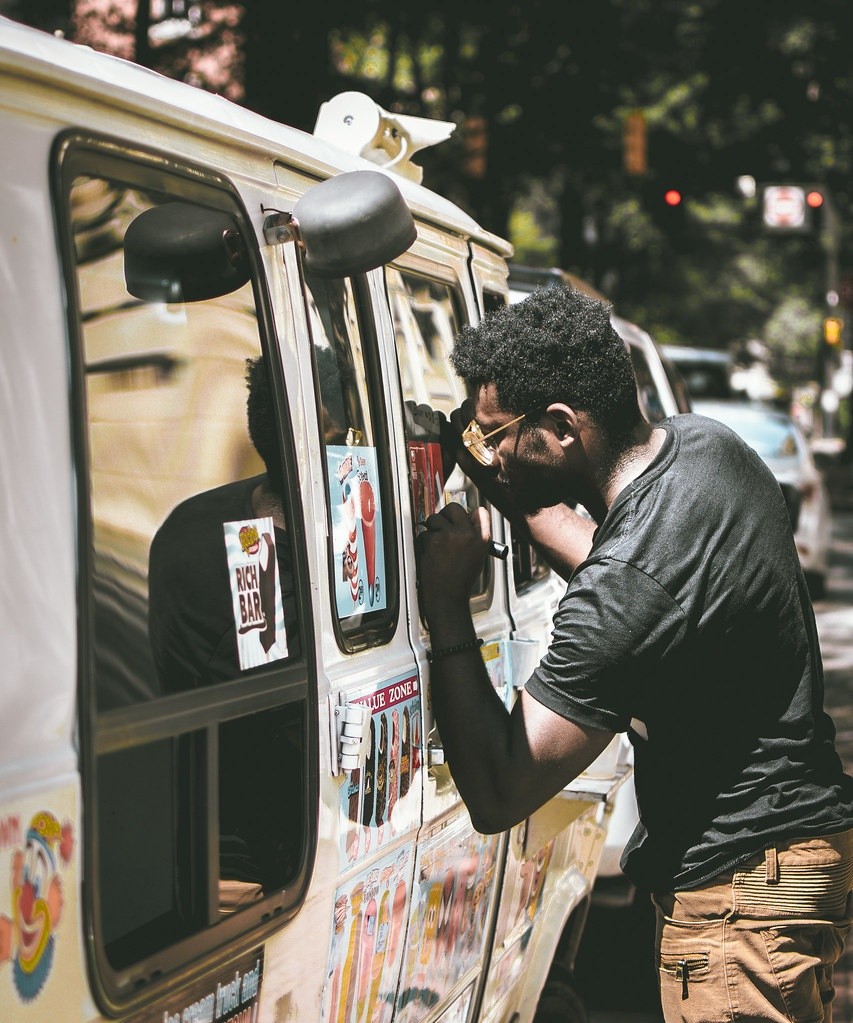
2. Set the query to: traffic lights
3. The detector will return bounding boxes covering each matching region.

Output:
[755,177,823,240]
[662,188,682,208]
[822,311,841,350]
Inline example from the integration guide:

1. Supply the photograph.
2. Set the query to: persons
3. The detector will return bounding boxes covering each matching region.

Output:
[147,345,451,933]
[412,285,853,1023]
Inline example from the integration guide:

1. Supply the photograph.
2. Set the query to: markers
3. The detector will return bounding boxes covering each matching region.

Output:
[420,520,509,561]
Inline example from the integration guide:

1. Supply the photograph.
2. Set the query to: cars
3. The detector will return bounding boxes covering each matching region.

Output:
[661,343,848,596]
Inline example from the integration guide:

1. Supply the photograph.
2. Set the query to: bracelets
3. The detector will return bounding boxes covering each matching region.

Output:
[425,636,483,660]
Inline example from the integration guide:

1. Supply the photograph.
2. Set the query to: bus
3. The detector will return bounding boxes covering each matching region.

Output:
[1,15,679,1023]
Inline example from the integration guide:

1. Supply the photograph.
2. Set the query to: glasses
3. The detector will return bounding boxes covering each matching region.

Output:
[461,402,579,466]
